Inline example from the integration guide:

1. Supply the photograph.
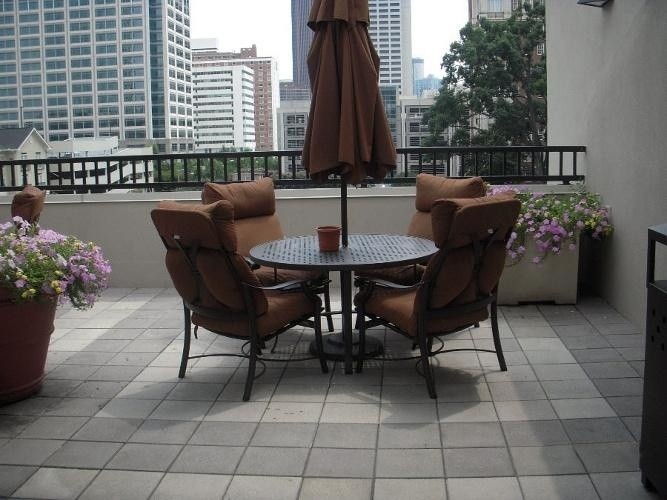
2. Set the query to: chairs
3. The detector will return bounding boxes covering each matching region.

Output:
[199,176,336,332]
[356,188,523,406]
[352,174,487,330]
[146,198,334,403]
[7,186,48,228]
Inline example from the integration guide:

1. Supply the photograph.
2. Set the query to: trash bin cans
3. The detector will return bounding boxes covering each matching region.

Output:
[639,223,667,500]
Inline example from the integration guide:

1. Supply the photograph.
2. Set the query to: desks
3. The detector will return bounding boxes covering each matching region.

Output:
[248,231,439,373]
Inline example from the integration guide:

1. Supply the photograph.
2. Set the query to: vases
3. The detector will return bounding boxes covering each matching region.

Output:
[494,225,585,309]
[0,287,59,407]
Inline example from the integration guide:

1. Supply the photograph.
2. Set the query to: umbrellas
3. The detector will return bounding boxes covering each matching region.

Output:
[302,0,398,246]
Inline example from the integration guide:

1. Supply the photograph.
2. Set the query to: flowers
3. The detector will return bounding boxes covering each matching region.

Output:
[484,182,615,263]
[0,215,111,315]
